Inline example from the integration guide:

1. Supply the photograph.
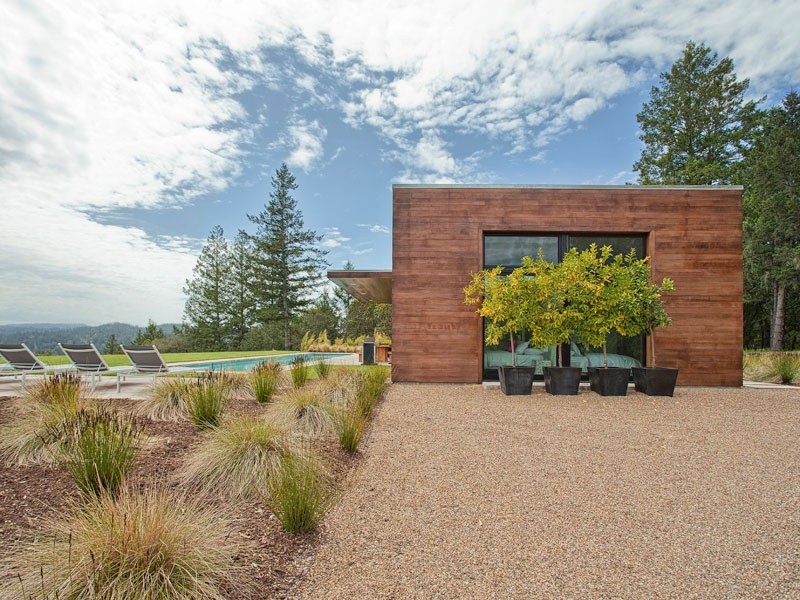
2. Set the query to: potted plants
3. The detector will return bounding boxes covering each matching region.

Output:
[462,243,680,397]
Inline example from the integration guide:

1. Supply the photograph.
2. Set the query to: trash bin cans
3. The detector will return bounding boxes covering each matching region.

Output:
[363,343,375,365]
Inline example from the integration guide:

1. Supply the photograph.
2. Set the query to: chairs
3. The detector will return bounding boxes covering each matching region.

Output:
[0,342,209,394]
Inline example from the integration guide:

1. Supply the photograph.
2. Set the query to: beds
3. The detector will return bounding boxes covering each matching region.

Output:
[484,349,544,368]
[571,353,643,376]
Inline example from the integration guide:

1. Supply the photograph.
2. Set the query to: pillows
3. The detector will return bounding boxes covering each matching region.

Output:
[515,341,531,354]
[570,351,575,356]
[502,339,516,351]
[571,341,581,356]
